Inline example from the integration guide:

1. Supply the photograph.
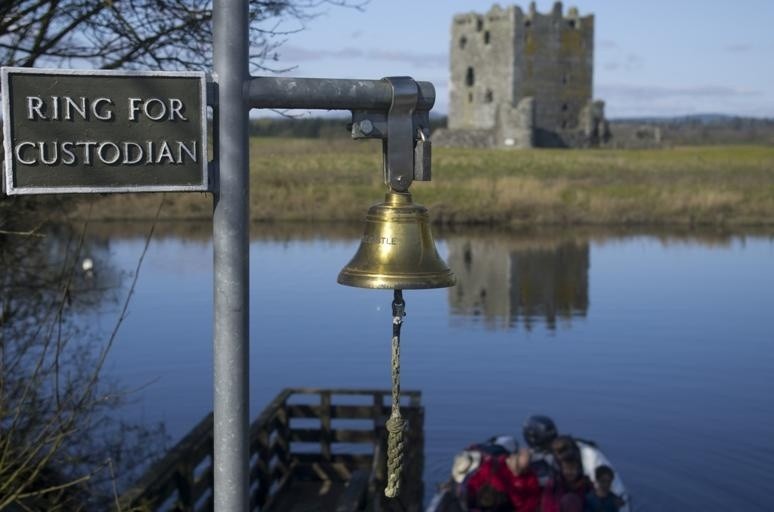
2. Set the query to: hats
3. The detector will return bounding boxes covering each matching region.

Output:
[451,449,481,484]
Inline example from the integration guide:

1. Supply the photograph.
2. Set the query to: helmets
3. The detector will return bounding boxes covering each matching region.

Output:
[522,415,558,451]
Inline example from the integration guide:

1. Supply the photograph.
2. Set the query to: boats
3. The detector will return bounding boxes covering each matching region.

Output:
[425,439,632,512]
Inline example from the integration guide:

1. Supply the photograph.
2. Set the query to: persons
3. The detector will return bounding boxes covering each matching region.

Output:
[448,441,626,512]
